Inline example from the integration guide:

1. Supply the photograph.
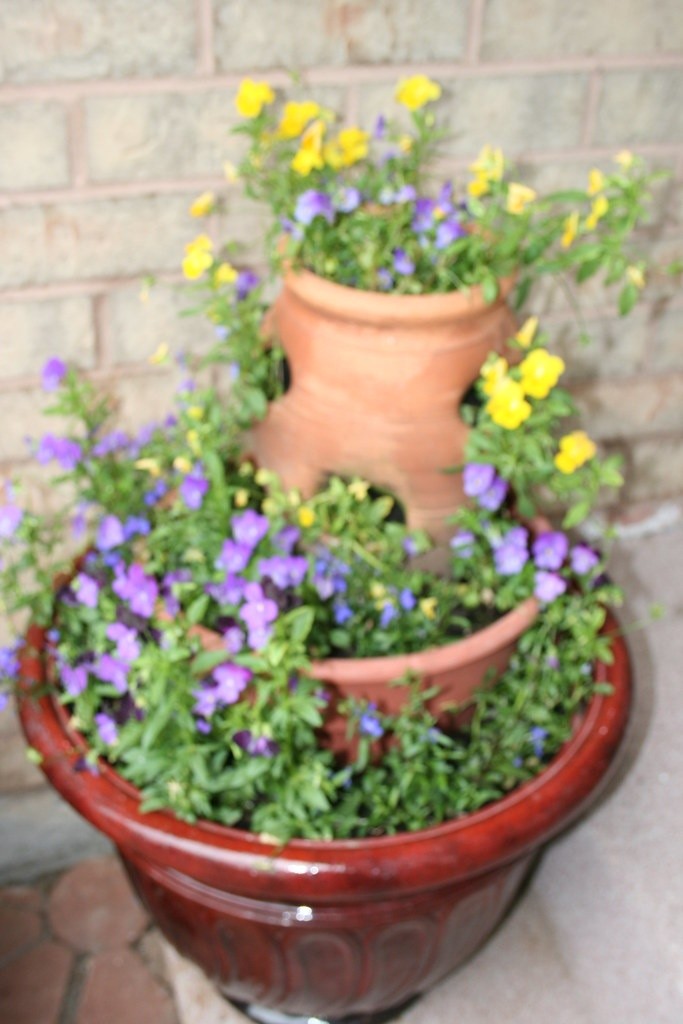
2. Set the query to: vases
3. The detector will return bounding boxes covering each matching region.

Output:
[17,542,636,1019]
[254,202,527,579]
[142,512,552,764]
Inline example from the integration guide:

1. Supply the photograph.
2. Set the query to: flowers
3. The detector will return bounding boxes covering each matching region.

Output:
[0,72,683,846]
